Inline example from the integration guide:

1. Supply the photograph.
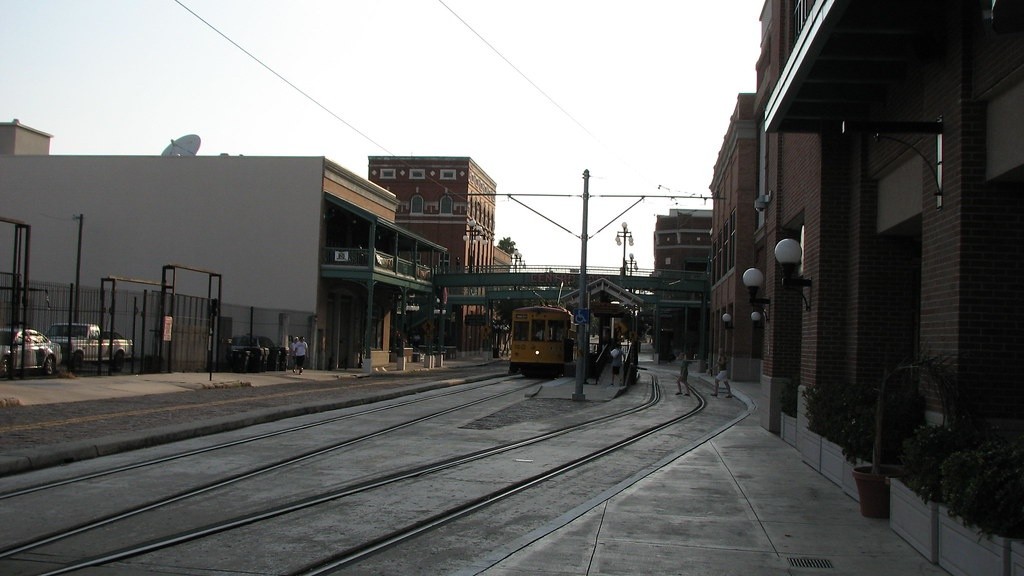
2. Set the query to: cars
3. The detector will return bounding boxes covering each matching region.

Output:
[0,328,63,377]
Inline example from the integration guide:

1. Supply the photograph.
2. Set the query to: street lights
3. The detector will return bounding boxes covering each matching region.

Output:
[614,220,639,292]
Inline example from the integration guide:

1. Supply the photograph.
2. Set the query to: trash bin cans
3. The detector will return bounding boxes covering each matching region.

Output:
[251,346,270,372]
[271,345,289,371]
[229,349,248,372]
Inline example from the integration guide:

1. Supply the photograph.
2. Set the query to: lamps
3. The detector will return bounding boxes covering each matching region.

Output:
[742,267,771,304]
[753,200,768,212]
[759,190,772,203]
[722,313,734,329]
[774,238,812,288]
[751,312,764,329]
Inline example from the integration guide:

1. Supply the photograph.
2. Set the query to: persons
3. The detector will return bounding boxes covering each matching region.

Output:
[608,344,626,386]
[295,335,309,375]
[289,336,299,373]
[674,353,692,396]
[710,346,733,399]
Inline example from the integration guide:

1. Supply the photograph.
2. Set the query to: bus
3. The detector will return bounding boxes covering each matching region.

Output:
[511,303,575,375]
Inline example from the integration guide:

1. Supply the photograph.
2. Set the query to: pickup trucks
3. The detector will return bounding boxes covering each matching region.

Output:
[46,322,132,372]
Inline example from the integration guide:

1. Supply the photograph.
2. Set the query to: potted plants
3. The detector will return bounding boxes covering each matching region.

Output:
[778,343,1024,576]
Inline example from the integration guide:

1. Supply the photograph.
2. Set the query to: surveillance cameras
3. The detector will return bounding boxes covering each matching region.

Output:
[754,197,769,212]
